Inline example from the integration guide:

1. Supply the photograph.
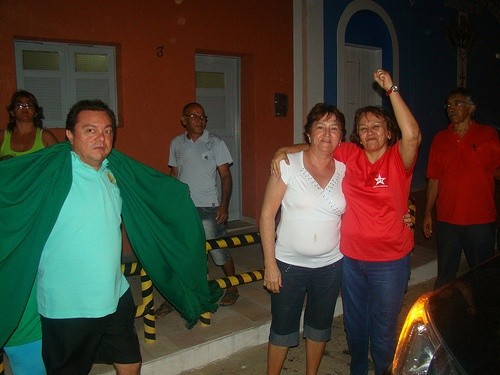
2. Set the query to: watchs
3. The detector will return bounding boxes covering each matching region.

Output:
[385,84,400,96]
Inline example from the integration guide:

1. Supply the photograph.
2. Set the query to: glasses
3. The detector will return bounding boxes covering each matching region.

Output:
[447,101,472,109]
[15,103,33,109]
[184,114,206,121]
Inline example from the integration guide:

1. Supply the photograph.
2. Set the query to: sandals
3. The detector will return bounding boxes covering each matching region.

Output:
[155,300,176,319]
[219,289,239,306]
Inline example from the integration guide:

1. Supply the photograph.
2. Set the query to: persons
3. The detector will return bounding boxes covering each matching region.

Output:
[423,88,500,333]
[259,102,347,375]
[0,91,221,375]
[153,102,240,319]
[271,68,420,375]
